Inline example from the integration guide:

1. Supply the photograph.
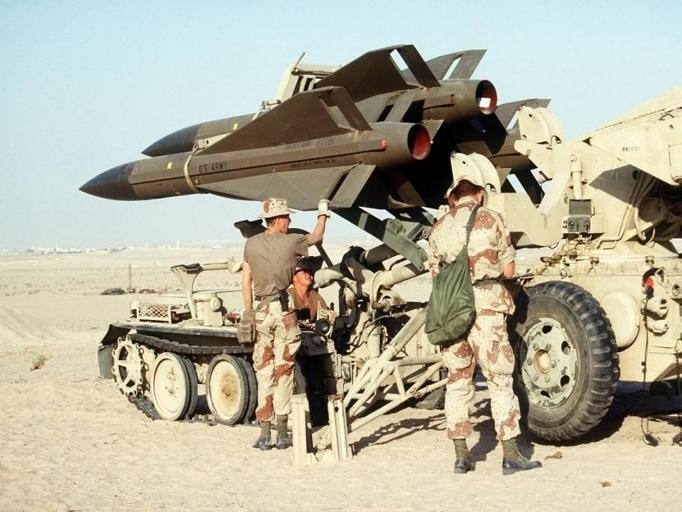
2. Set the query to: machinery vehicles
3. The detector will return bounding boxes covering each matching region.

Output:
[78,41,682,447]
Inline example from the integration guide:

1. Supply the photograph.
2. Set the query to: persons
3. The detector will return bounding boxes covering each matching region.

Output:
[237,197,331,451]
[429,174,542,477]
[292,259,336,431]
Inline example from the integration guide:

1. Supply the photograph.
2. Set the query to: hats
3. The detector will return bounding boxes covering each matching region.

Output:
[446,176,488,206]
[258,198,295,219]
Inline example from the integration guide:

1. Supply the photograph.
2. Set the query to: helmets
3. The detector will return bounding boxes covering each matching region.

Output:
[293,259,314,274]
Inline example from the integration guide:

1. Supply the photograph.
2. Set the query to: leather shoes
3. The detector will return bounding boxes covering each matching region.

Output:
[454,457,475,474]
[254,437,273,450]
[501,455,540,474]
[277,433,292,449]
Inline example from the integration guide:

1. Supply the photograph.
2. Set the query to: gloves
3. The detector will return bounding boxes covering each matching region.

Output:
[316,199,331,218]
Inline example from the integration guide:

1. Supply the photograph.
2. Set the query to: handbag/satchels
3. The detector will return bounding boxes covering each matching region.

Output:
[424,245,475,345]
